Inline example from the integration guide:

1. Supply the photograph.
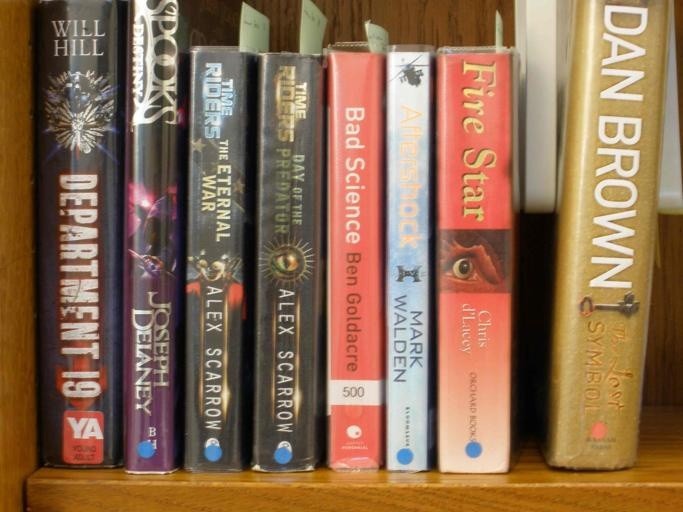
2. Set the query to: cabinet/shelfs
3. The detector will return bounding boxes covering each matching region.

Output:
[0,1,682,512]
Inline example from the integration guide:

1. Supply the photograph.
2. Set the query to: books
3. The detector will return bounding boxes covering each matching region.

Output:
[248,51,323,474]
[322,49,387,471]
[181,45,254,476]
[383,45,433,472]
[434,43,514,472]
[542,0,669,470]
[121,0,188,476]
[29,1,120,470]
